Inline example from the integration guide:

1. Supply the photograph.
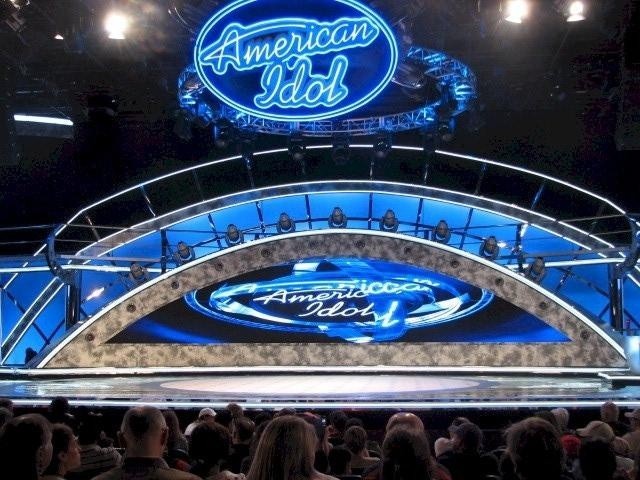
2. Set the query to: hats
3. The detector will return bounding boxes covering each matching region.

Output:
[561,435,581,455]
[199,408,218,417]
[448,424,483,441]
[576,421,613,442]
[625,408,640,419]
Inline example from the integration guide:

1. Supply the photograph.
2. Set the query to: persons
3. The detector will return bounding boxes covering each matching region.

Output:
[1,397,640,480]
